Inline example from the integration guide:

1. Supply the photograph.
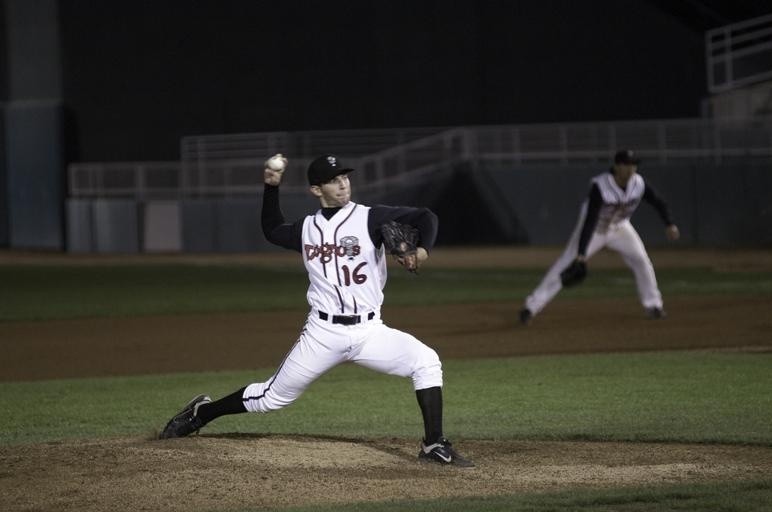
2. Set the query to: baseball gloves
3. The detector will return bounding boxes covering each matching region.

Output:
[380,221,423,275]
[560,258,587,288]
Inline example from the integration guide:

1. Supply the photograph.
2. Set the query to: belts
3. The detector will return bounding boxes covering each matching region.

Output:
[318,311,375,324]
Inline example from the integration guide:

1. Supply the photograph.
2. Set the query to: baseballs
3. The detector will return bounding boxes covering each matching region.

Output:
[268,156,284,170]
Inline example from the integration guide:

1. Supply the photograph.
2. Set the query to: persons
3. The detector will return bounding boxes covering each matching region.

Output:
[520,149,680,326]
[159,155,475,468]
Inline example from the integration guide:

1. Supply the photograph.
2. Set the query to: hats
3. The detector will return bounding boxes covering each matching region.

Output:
[616,150,640,164]
[308,154,353,185]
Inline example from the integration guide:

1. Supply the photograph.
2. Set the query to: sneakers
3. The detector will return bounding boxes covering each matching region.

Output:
[519,308,530,324]
[648,308,666,318]
[163,393,212,438]
[418,436,475,468]
